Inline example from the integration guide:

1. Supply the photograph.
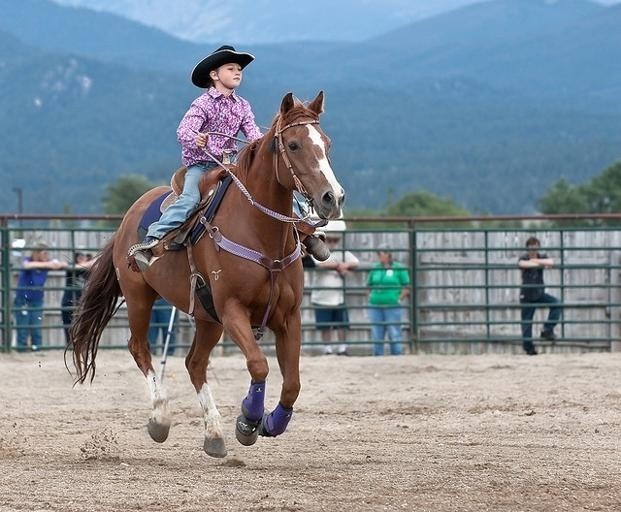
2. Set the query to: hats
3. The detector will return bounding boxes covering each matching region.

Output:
[191,45,255,89]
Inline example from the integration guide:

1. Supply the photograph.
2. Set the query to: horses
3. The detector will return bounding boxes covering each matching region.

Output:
[64,90,347,459]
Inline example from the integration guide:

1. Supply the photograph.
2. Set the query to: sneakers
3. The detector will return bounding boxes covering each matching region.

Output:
[302,235,331,262]
[134,249,152,273]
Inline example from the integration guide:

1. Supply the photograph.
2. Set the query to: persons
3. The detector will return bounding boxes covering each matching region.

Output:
[123,42,330,271]
[13,241,67,351]
[144,294,180,355]
[366,244,415,357]
[59,251,101,347]
[515,236,565,357]
[307,222,361,357]
